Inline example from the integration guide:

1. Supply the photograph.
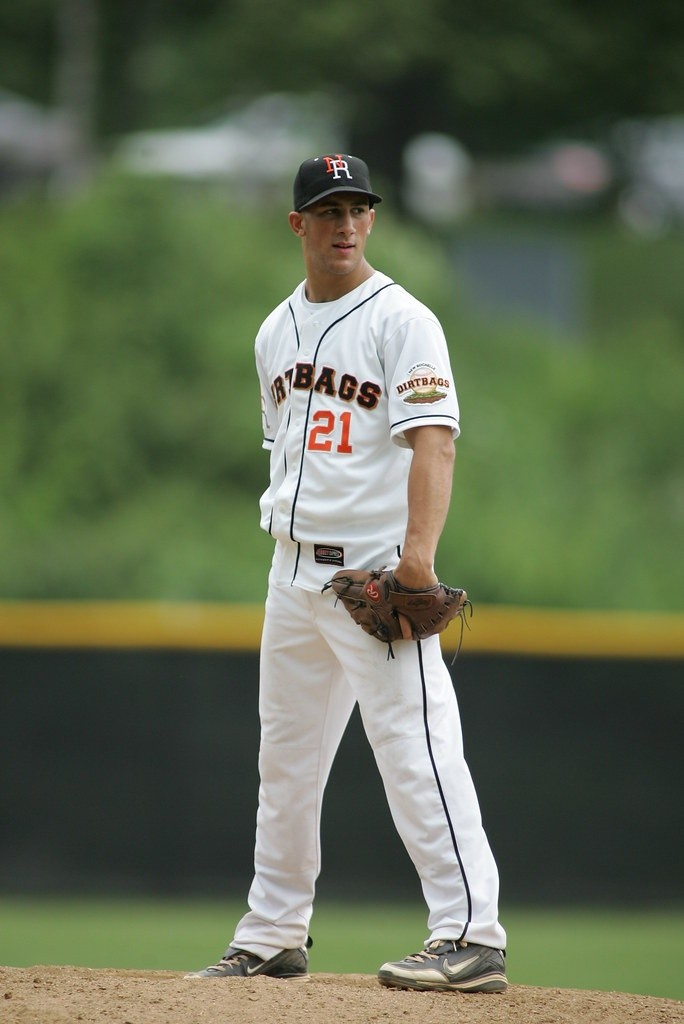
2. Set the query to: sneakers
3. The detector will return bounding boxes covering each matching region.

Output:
[185,938,309,979]
[378,938,509,991]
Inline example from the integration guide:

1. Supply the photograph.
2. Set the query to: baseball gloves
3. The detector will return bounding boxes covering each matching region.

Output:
[325,562,471,648]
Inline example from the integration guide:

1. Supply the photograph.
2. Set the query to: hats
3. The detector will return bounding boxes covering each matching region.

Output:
[292,153,383,213]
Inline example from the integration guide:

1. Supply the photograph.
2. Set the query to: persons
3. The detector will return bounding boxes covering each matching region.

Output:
[189,153,511,992]
